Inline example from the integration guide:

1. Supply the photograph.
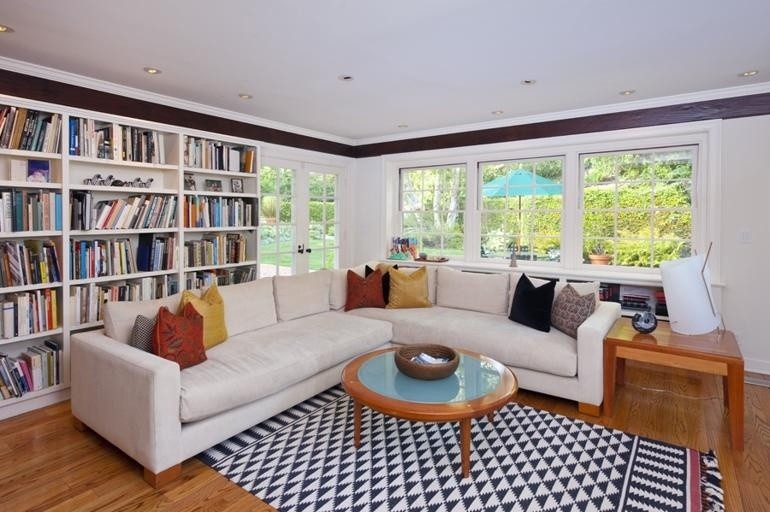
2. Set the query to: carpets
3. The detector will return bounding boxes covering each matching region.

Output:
[193,381,726,511]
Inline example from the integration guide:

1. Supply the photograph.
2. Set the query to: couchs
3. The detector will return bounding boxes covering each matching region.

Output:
[69,258,623,491]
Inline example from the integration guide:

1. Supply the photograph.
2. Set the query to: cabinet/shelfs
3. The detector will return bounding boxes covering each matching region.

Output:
[0,90,70,426]
[66,104,179,403]
[179,124,262,292]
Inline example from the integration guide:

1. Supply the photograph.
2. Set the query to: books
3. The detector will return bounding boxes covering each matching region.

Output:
[183,195,257,291]
[621,293,652,312]
[69,118,165,164]
[597,283,620,302]
[184,135,255,173]
[70,191,179,326]
[654,290,669,315]
[393,234,418,259]
[1,105,62,400]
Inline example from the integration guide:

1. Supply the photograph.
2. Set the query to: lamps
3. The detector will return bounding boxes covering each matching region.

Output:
[659,241,726,337]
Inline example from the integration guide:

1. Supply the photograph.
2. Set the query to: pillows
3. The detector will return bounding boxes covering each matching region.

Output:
[386,265,433,308]
[436,264,510,317]
[344,268,385,312]
[152,300,209,372]
[551,282,597,341]
[175,279,229,350]
[508,270,601,316]
[398,267,437,305]
[508,272,557,333]
[363,264,401,305]
[129,314,157,353]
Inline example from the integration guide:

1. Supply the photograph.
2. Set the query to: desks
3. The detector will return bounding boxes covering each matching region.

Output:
[603,315,746,454]
[563,268,670,321]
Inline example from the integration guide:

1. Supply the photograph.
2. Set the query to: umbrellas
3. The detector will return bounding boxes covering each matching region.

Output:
[484,168,563,255]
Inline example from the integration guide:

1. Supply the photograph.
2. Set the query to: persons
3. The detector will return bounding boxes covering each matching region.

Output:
[234,181,242,192]
[207,181,222,192]
[184,175,196,191]
